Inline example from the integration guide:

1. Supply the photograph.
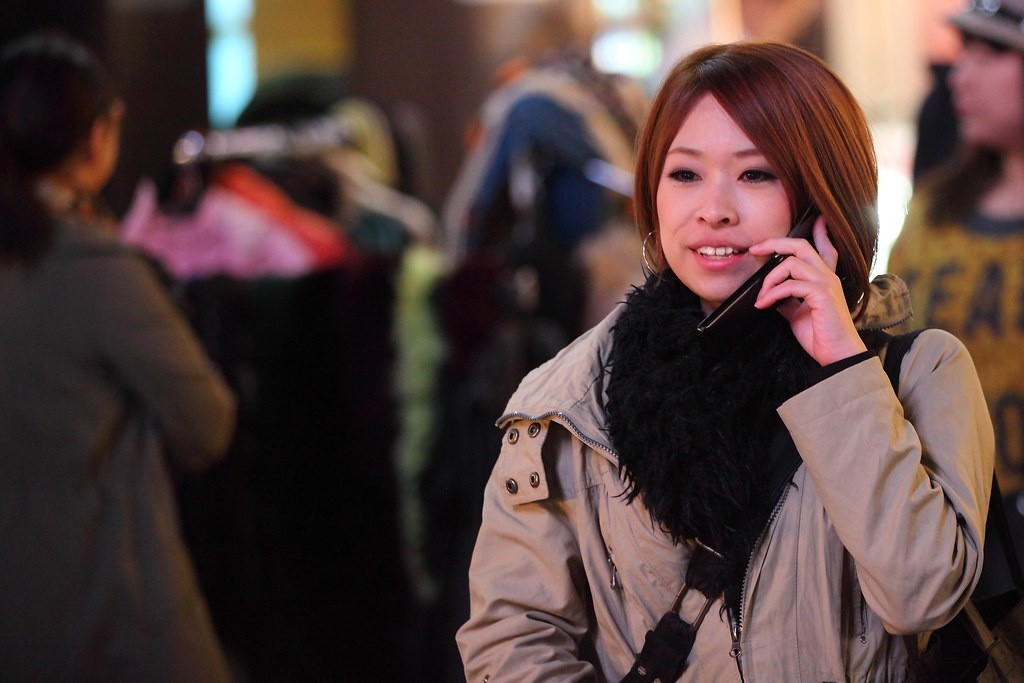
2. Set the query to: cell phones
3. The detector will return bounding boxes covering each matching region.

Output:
[698,203,830,348]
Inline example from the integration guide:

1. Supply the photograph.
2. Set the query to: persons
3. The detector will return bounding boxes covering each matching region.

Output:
[455,43,996,683]
[130,62,652,682]
[911,57,965,204]
[0,29,241,682]
[883,1,1024,557]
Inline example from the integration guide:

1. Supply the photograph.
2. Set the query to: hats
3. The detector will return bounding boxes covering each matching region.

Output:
[947,0,1024,51]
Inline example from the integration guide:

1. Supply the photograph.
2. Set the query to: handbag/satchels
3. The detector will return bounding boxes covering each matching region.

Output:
[909,593,1024,683]
